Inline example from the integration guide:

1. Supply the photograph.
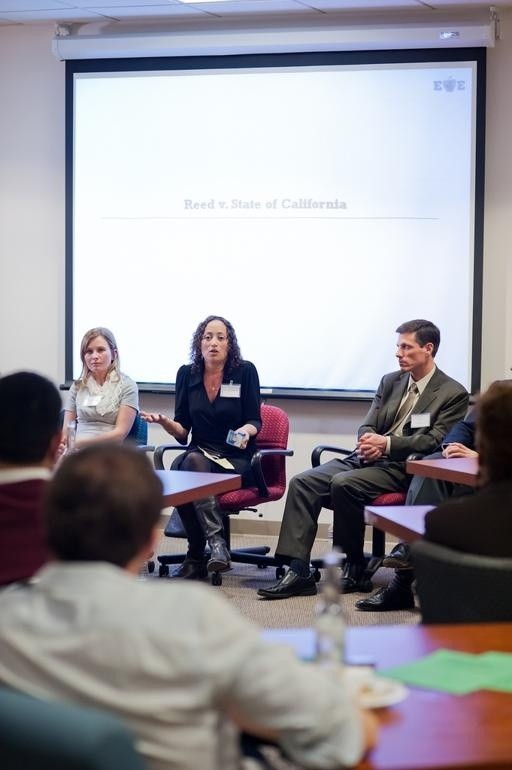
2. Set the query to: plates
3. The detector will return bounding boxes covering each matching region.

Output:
[300,662,409,713]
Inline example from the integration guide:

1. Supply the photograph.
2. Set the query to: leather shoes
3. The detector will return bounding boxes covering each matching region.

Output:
[334,551,366,593]
[354,585,417,613]
[381,541,413,568]
[257,569,316,598]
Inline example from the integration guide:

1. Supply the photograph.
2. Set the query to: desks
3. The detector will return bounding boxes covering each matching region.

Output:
[149,464,262,519]
[263,620,510,768]
[364,499,438,545]
[406,457,479,491]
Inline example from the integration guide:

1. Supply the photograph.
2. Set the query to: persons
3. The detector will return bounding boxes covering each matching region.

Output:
[412,377,512,628]
[0,437,382,770]
[353,368,512,612]
[0,371,64,589]
[255,317,470,602]
[56,326,140,459]
[140,314,263,583]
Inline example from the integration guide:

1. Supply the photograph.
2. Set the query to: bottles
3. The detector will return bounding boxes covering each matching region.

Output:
[65,423,80,460]
[314,545,350,664]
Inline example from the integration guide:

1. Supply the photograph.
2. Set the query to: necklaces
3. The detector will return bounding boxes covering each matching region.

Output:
[204,372,221,392]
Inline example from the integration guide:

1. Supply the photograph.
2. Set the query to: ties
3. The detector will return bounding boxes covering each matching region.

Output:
[381,383,419,438]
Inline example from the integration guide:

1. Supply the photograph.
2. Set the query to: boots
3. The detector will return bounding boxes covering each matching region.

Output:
[161,506,209,580]
[195,495,233,572]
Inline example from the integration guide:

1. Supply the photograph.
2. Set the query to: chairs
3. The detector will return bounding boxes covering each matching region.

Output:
[149,399,300,576]
[61,406,157,450]
[300,442,404,588]
